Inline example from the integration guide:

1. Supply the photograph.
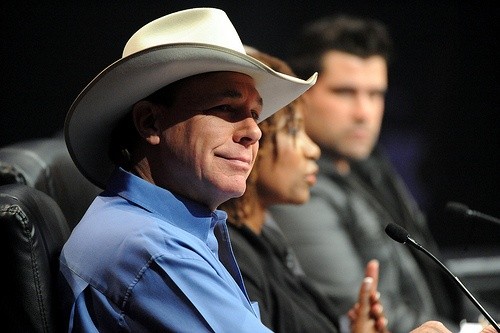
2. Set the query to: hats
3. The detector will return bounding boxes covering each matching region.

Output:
[64,7,318,191]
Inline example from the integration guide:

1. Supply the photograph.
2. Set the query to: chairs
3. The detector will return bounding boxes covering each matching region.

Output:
[0,138,104,333]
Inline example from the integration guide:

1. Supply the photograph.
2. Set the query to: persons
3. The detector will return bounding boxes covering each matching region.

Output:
[215,44,389,333]
[268,17,500,333]
[57,5,455,333]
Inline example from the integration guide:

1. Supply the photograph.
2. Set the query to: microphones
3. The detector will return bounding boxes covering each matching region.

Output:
[385,223,500,333]
[441,201,500,224]
